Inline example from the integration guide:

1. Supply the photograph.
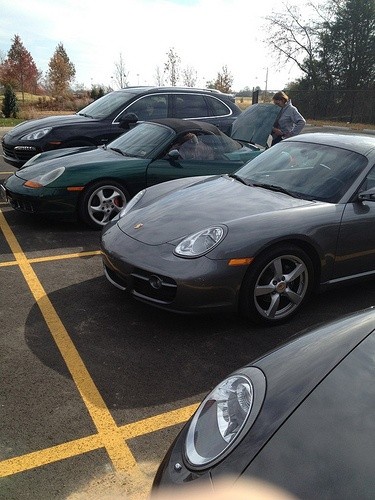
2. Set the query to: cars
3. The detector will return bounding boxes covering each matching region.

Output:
[3,87,240,169]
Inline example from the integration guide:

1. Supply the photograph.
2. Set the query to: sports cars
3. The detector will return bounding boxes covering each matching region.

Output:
[101,132,375,323]
[7,102,285,227]
[151,308,375,500]
